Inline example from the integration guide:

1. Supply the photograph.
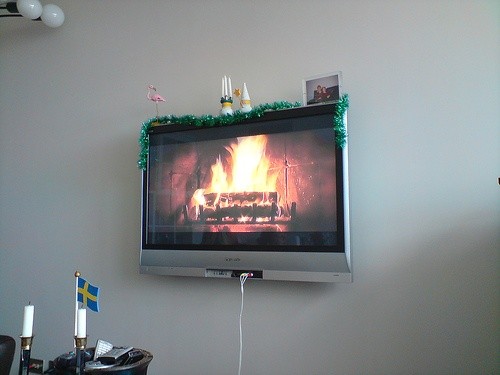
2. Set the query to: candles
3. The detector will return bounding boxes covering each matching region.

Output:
[22,300,34,337]
[228,77,232,97]
[77,305,86,338]
[225,75,227,96]
[222,77,224,97]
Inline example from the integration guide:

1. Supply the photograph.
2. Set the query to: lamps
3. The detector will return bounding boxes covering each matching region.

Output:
[0,0,42,19]
[0,4,65,28]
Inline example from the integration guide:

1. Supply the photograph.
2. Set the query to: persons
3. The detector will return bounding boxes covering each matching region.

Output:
[313,86,322,102]
[321,87,331,100]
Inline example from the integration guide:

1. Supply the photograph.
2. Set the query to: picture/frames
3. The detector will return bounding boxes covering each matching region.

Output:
[303,71,342,107]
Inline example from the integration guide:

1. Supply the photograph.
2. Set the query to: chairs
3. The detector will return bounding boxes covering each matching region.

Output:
[0,335,16,375]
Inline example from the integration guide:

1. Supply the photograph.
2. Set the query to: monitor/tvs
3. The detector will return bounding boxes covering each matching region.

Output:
[139,103,353,283]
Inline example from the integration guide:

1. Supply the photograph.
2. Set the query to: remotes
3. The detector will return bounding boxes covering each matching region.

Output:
[93,340,113,363]
[98,346,134,363]
